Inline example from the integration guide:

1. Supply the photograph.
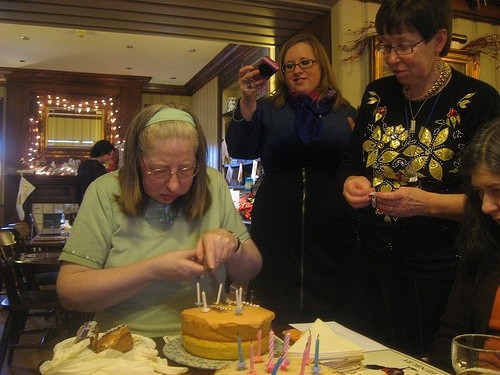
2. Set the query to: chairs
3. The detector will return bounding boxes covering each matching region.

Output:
[0,222,74,375]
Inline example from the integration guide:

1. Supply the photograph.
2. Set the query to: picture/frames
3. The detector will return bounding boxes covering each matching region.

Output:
[371,35,481,84]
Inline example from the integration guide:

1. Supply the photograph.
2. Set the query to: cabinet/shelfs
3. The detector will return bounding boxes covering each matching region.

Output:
[220,48,269,222]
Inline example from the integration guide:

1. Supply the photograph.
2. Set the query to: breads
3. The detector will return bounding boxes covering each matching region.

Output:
[87,321,136,353]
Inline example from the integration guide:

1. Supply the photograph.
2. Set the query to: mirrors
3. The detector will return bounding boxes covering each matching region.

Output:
[39,96,111,158]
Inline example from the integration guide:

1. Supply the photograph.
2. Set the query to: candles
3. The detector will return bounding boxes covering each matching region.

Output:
[235,329,320,375]
[195,281,243,312]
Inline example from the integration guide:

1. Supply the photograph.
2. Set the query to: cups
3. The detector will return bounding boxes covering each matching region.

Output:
[450,334,500,375]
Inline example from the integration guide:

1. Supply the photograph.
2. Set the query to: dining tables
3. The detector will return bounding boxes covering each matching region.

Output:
[66,319,456,375]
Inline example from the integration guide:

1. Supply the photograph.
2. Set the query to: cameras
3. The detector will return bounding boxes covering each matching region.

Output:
[248,56,279,82]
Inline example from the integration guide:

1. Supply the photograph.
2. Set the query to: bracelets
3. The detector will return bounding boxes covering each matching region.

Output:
[229,230,241,252]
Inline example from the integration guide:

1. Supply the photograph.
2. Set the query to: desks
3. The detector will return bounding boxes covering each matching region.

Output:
[31,227,71,251]
[13,251,83,345]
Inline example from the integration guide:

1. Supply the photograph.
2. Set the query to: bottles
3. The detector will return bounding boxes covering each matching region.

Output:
[226,97,237,112]
[60,214,65,230]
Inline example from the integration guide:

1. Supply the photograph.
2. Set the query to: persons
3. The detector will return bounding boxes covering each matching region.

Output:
[56,103,262,338]
[343,0,500,375]
[77,140,115,202]
[224,33,358,323]
[427,118,500,375]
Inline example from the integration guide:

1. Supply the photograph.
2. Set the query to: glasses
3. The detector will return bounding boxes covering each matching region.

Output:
[140,154,199,181]
[282,58,317,73]
[375,37,424,55]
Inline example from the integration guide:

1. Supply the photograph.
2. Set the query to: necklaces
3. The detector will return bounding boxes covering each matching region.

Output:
[402,63,451,133]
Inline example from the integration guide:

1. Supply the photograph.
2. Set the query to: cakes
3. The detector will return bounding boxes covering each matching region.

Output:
[211,355,341,375]
[181,302,275,359]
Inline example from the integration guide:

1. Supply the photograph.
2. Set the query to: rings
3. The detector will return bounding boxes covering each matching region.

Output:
[391,207,392,211]
[251,82,254,87]
